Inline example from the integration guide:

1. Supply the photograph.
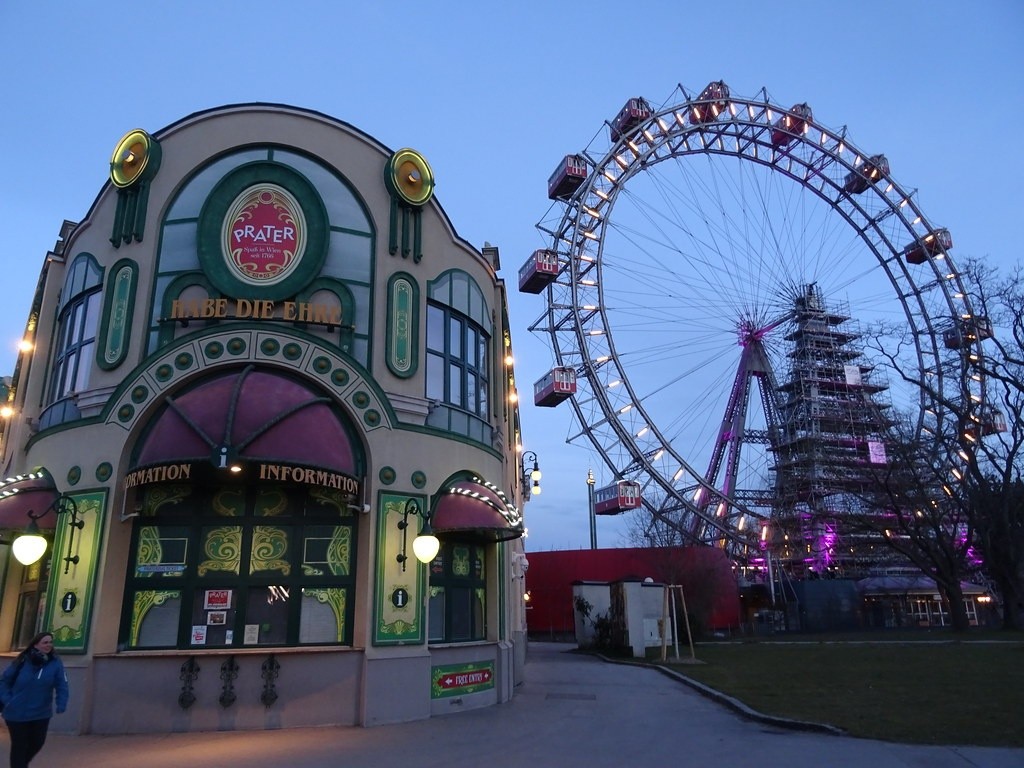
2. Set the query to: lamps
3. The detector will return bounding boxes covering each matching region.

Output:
[396,497,441,572]
[13,496,84,574]
[520,449,542,502]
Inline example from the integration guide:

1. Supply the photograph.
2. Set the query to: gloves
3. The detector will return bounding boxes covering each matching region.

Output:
[56,704,66,713]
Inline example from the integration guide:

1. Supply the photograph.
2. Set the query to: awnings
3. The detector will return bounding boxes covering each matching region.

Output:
[0,473,59,529]
[429,474,525,543]
[121,363,360,500]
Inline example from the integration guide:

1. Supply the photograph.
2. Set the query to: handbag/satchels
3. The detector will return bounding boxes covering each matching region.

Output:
[0,655,25,713]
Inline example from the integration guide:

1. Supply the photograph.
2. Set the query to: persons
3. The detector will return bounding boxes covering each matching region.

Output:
[0,632,69,768]
